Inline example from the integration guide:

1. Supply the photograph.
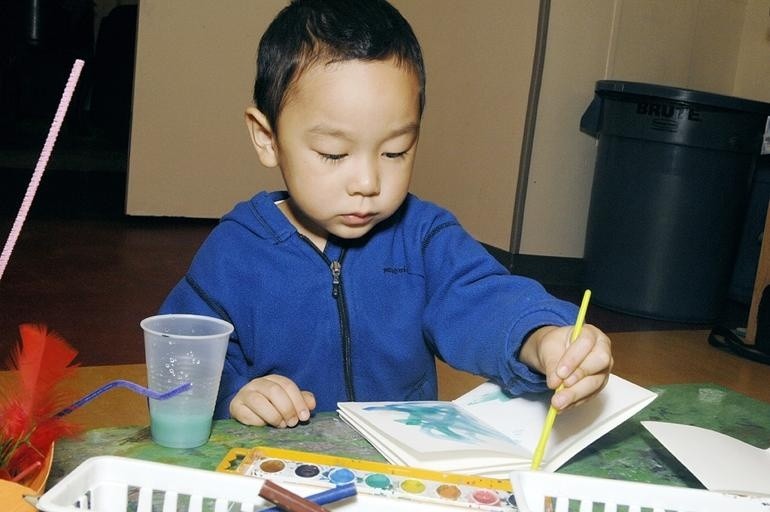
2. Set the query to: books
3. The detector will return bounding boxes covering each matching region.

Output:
[331,362,659,482]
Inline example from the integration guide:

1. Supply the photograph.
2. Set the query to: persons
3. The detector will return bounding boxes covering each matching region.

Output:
[152,0,614,427]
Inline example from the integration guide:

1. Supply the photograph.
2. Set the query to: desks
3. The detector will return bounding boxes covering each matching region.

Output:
[2,326,770,512]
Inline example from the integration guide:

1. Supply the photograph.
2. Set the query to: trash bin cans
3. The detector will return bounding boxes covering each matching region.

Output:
[585,78,768,332]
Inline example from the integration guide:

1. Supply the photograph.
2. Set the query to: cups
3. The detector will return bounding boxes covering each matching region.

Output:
[139,312,234,451]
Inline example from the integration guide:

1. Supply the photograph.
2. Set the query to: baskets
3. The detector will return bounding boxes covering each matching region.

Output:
[509,469,729,512]
[37,455,325,512]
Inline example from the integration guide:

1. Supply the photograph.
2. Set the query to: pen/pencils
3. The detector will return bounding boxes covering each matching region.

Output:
[528,289,593,472]
